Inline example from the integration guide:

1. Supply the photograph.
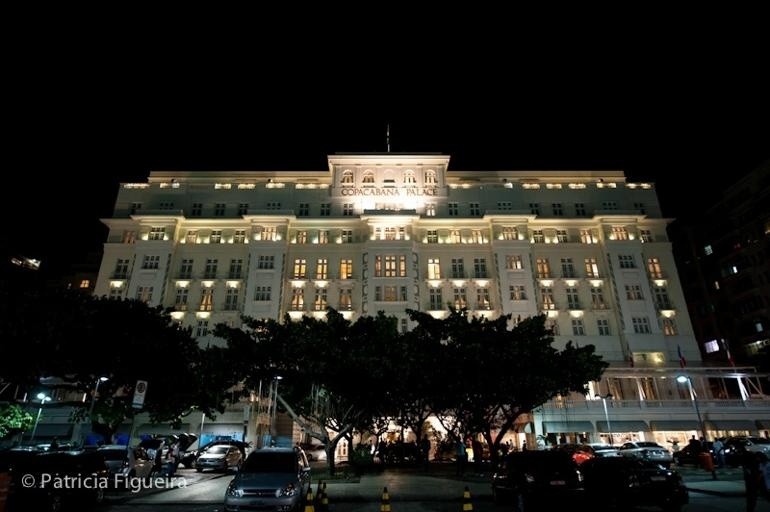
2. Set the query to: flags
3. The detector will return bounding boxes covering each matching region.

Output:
[676,345,686,371]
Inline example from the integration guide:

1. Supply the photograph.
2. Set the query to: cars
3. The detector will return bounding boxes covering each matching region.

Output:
[485,435,770,511]
[0,442,241,491]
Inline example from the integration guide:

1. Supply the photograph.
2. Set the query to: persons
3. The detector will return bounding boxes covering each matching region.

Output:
[594,394,602,401]
[371,428,484,478]
[690,435,725,469]
[155,441,181,483]
[522,440,526,451]
[50,437,59,451]
[506,439,513,449]
[672,441,679,462]
[300,442,305,452]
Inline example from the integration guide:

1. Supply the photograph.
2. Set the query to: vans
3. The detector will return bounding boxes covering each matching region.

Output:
[269,374,285,445]
[220,445,315,511]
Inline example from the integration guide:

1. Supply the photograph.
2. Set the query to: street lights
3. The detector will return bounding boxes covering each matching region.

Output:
[28,389,56,454]
[595,394,616,446]
[673,374,707,448]
